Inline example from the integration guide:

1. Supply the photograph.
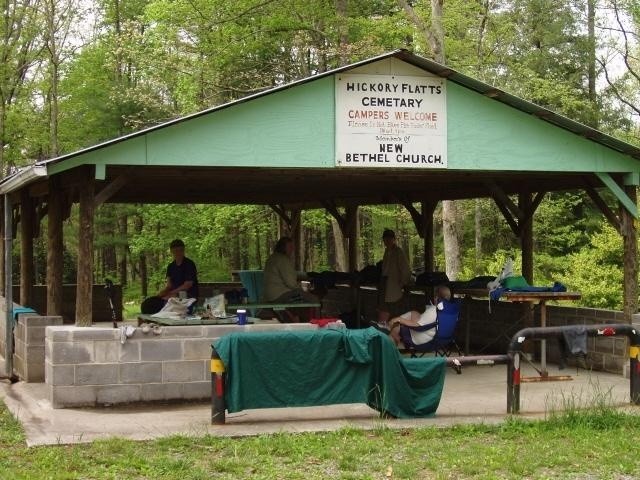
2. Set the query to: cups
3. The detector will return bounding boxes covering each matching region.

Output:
[237,310,246,325]
[301,279,309,292]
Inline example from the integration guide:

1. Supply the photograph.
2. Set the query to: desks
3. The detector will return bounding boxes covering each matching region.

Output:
[137,314,254,326]
[409,285,582,382]
[195,303,321,323]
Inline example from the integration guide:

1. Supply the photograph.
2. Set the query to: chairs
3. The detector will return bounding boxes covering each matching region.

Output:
[394,297,463,358]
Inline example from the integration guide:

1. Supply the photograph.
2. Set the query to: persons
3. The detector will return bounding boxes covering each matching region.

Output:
[140,239,198,318]
[223,273,248,305]
[262,237,322,323]
[376,228,411,324]
[366,286,451,348]
